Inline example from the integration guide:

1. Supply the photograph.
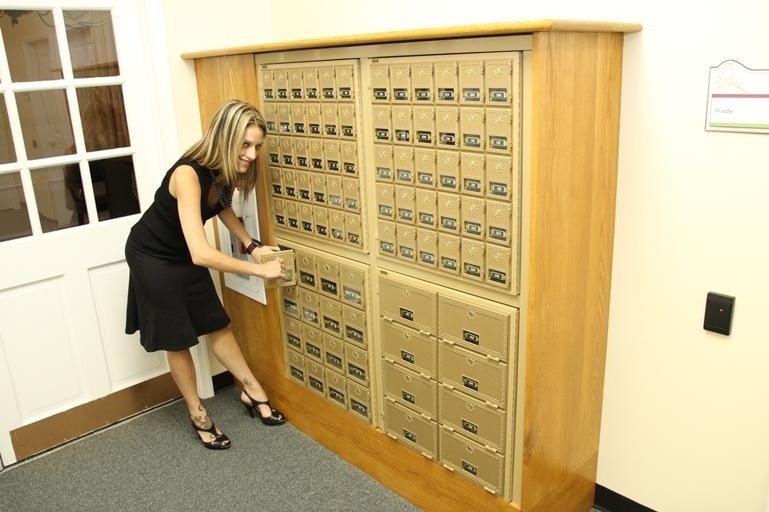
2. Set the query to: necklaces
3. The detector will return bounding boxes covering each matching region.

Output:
[210,168,233,209]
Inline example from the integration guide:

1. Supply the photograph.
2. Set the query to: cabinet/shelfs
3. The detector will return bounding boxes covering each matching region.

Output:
[48,60,138,200]
[177,20,644,512]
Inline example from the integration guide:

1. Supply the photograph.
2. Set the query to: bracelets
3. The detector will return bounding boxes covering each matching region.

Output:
[246,242,258,254]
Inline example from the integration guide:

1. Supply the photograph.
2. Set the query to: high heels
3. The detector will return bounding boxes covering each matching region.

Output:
[191,418,232,450]
[240,389,286,426]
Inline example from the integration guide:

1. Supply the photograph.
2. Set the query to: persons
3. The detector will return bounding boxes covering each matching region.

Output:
[62,94,141,226]
[123,98,291,450]
